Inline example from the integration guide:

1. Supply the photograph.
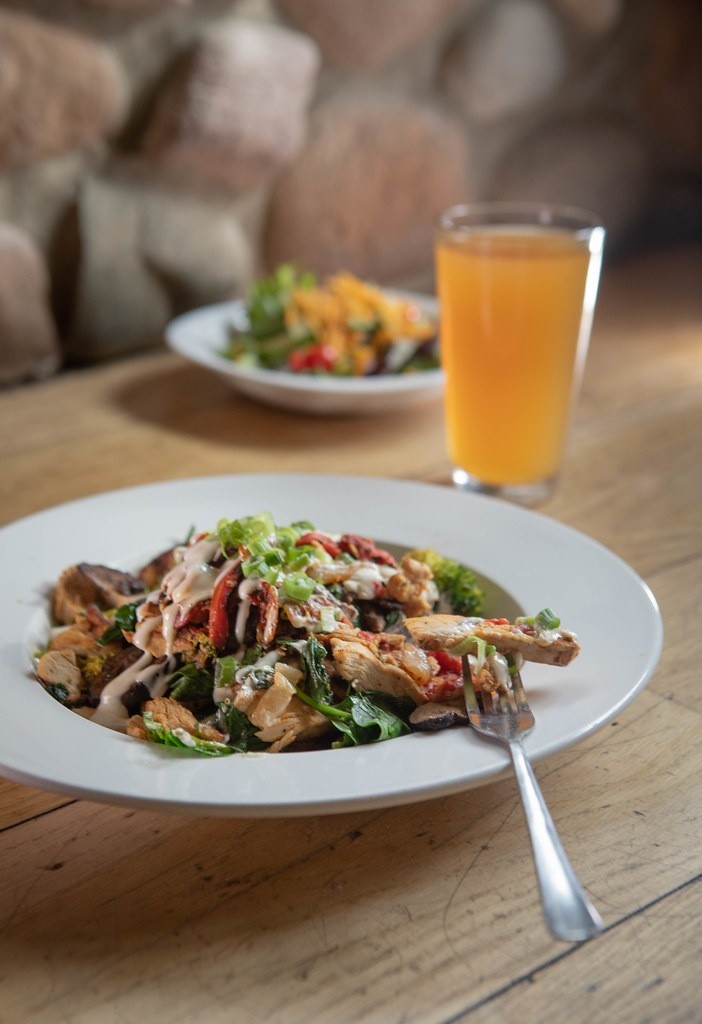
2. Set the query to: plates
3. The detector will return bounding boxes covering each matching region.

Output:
[165,287,449,418]
[0,470,665,820]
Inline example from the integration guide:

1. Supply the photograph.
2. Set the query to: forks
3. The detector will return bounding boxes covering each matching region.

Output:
[461,649,604,943]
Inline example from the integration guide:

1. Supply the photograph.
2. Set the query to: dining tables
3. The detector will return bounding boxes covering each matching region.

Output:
[0,245,701,1024]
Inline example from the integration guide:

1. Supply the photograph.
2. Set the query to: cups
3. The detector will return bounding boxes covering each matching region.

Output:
[435,200,606,507]
